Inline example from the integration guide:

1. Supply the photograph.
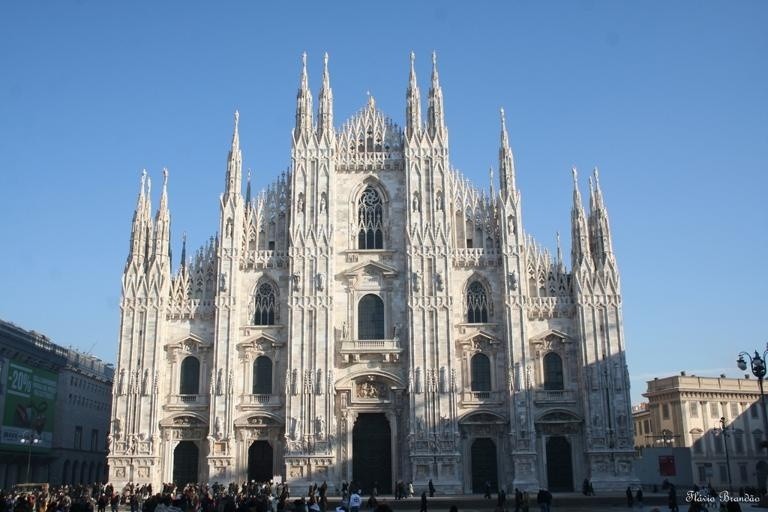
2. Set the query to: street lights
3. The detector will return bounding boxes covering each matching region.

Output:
[713,417,735,497]
[20,431,42,482]
[656,430,675,448]
[736,349,767,441]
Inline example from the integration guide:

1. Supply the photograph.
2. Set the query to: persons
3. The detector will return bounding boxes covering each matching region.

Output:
[0,477,435,512]
[483,477,553,512]
[624,481,768,512]
